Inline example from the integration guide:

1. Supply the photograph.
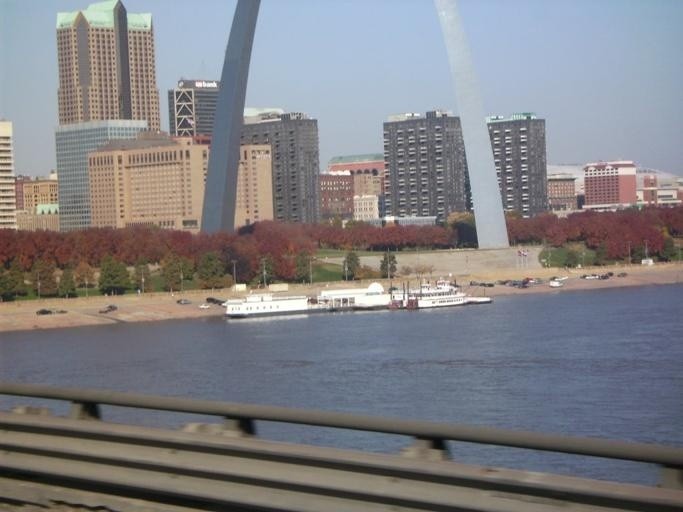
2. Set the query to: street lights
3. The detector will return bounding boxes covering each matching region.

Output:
[262,265,268,287]
[140,268,145,294]
[231,259,238,284]
[387,257,391,278]
[309,260,313,284]
[344,262,349,282]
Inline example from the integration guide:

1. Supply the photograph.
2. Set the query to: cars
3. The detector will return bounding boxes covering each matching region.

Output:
[470,271,627,287]
[36,297,225,316]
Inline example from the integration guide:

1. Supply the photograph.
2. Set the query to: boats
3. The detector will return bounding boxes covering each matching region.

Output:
[391,277,467,308]
